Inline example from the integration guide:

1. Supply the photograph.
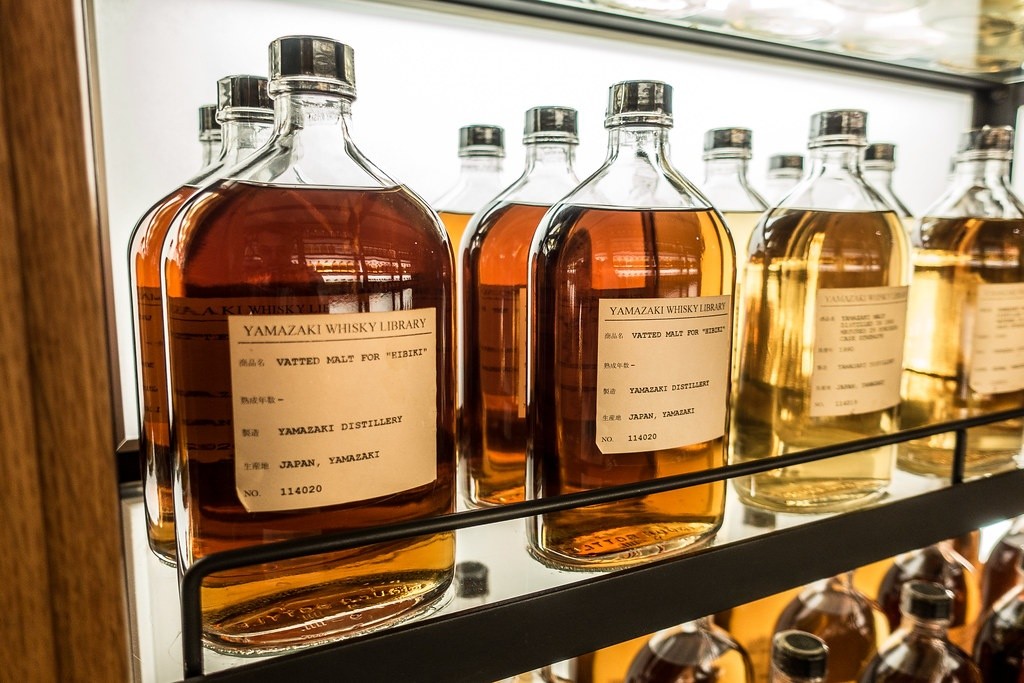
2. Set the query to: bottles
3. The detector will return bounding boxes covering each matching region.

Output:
[429,122,510,255]
[899,125,1024,478]
[448,501,977,683]
[157,36,457,661]
[764,154,804,206]
[125,74,283,566]
[697,130,774,452]
[864,142,924,436]
[969,583,1024,683]
[462,106,581,514]
[733,109,912,514]
[527,80,734,573]
[979,513,1024,613]
